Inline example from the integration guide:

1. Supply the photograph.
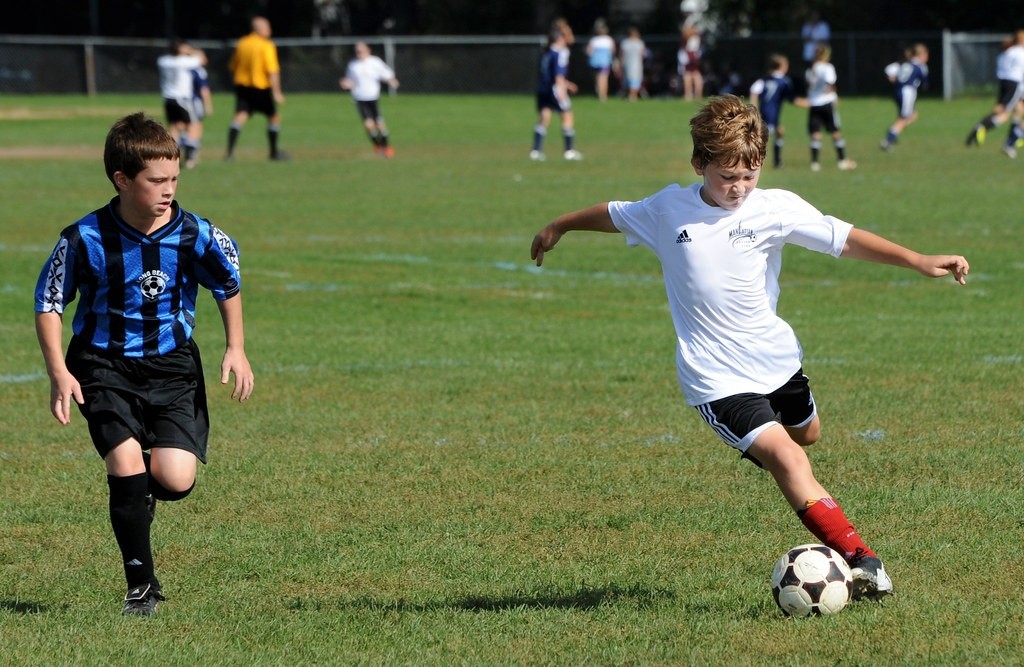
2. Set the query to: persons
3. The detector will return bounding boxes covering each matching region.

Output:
[155,38,216,173]
[878,44,931,149]
[674,0,857,171]
[528,17,652,164]
[965,30,1024,159]
[34,111,254,614]
[227,16,292,164]
[339,40,401,156]
[530,93,969,599]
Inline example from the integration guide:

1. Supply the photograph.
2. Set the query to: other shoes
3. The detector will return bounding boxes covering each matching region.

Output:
[374,143,394,158]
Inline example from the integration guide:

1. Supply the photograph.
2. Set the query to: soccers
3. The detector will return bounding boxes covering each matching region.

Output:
[772,544,854,616]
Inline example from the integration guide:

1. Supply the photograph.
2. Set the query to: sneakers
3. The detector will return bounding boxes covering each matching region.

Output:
[122,582,159,617]
[845,546,894,597]
